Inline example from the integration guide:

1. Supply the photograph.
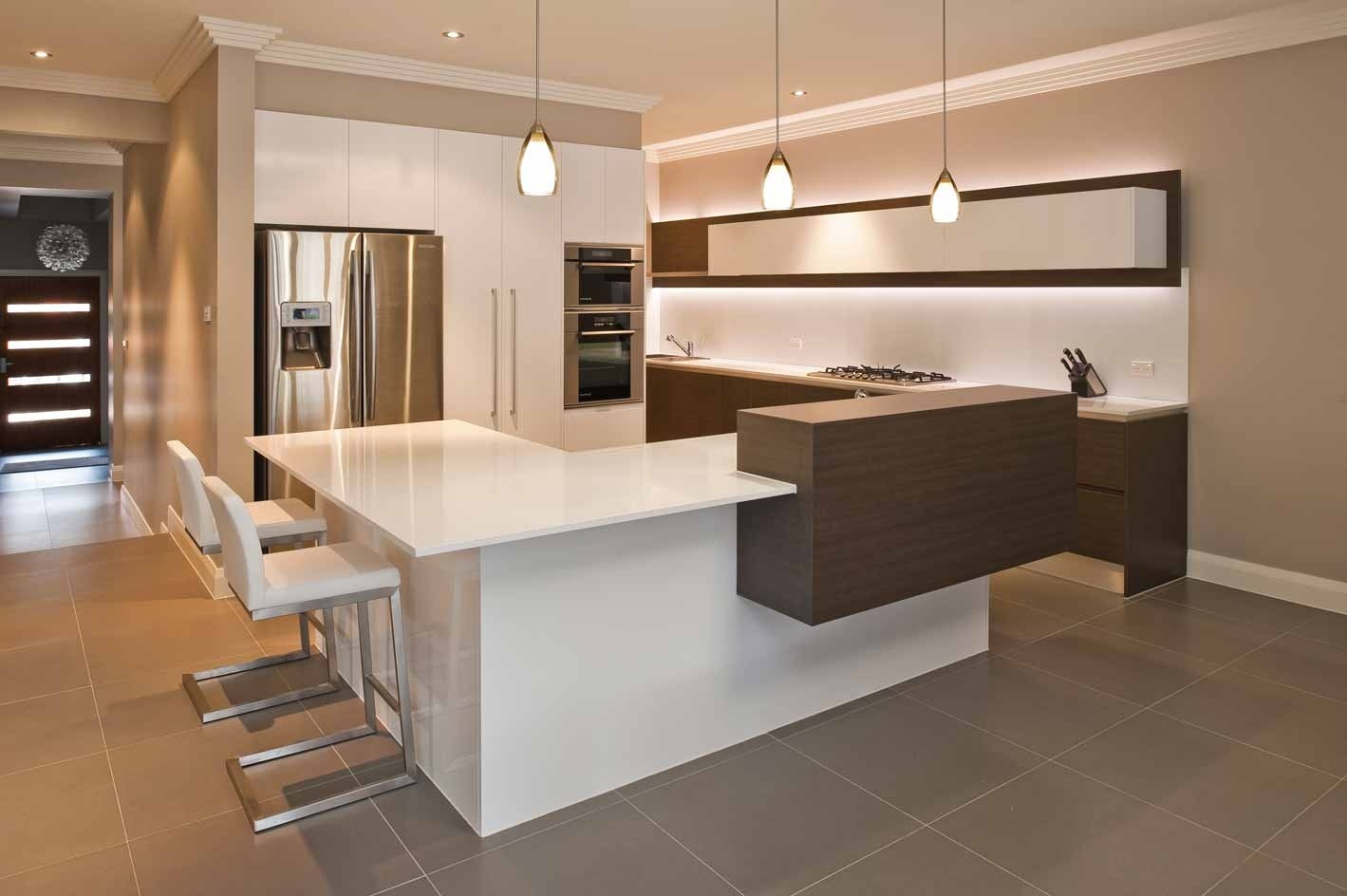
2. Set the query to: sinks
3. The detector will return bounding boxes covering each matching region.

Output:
[646,356,711,362]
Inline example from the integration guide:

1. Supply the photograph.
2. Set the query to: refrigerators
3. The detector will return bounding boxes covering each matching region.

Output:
[255,226,443,554]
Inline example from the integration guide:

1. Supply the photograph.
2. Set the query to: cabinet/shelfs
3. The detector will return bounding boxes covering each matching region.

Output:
[647,368,1189,600]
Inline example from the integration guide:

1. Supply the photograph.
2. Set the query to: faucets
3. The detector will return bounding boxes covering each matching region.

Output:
[666,335,693,357]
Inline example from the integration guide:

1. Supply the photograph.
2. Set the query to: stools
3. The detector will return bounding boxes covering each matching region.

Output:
[167,439,417,834]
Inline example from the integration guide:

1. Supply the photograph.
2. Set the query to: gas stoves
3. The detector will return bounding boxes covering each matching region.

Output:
[807,364,958,388]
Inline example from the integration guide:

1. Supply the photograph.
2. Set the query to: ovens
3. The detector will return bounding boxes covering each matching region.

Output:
[564,242,644,410]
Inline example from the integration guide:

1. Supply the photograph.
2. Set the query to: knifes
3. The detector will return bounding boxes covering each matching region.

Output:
[1061,348,1088,375]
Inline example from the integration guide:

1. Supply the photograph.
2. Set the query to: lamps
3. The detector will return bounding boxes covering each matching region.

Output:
[760,0,796,212]
[515,0,560,197]
[929,0,962,222]
